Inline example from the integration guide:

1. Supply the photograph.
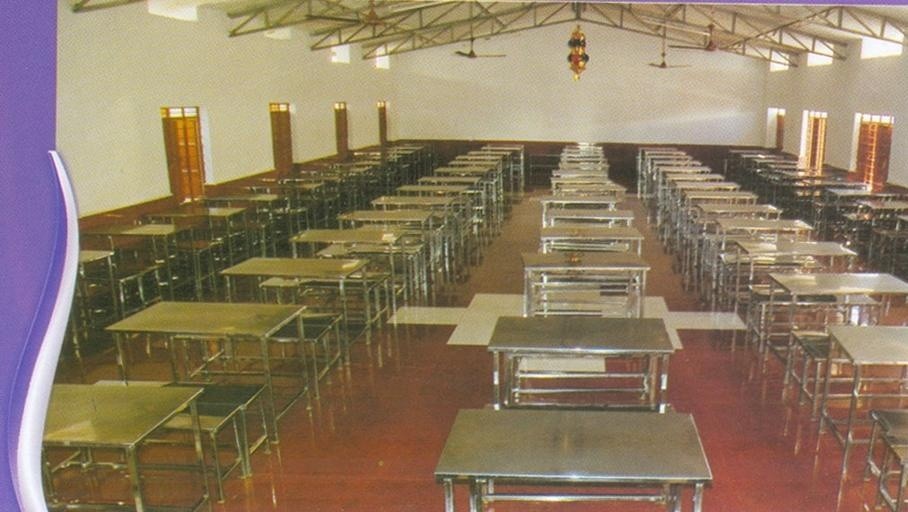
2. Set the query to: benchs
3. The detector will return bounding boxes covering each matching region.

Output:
[176,324,333,401]
[90,382,268,479]
[85,262,164,319]
[115,402,248,503]
[719,252,828,308]
[126,238,226,299]
[788,328,908,421]
[862,407,908,511]
[284,312,344,386]
[259,272,392,334]
[481,400,675,413]
[747,284,881,354]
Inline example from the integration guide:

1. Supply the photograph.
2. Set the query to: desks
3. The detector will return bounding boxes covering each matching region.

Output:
[66,249,121,367]
[433,407,715,512]
[540,195,620,227]
[289,228,409,314]
[371,195,472,259]
[734,239,858,326]
[520,250,651,318]
[204,193,284,256]
[102,300,313,444]
[538,225,644,257]
[681,189,761,249]
[83,224,199,303]
[548,208,635,227]
[147,203,252,266]
[763,271,908,384]
[817,324,908,461]
[244,182,329,229]
[559,184,626,199]
[41,383,214,512]
[218,257,373,365]
[337,208,436,297]
[485,315,675,405]
[695,202,782,279]
[711,216,816,291]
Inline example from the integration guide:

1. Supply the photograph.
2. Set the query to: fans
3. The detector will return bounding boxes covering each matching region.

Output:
[305,1,411,33]
[668,22,749,54]
[455,1,507,60]
[648,22,695,70]
